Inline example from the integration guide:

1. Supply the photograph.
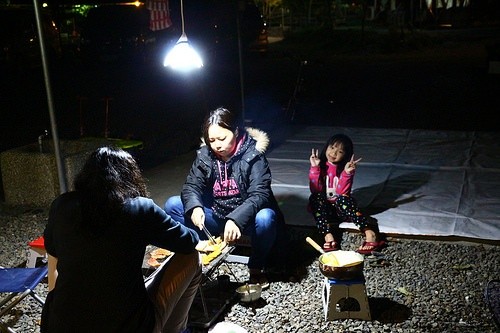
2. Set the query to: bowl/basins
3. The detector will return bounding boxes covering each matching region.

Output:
[235,284,263,302]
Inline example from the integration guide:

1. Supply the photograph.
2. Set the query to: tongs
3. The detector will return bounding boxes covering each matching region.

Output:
[201,223,217,245]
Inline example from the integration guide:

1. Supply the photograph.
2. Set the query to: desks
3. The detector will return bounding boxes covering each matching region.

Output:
[140,241,236,331]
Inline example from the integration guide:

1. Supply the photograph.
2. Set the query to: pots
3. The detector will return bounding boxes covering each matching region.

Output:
[306,236,364,281]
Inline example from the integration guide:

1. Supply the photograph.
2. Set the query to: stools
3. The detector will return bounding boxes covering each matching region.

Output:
[322,272,371,321]
[24,237,47,269]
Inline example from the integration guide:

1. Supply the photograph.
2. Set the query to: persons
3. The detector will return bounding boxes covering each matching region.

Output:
[40,145,202,333]
[164,106,288,289]
[309,133,385,254]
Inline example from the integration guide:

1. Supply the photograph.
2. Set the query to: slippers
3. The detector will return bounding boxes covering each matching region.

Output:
[322,240,337,251]
[357,240,385,254]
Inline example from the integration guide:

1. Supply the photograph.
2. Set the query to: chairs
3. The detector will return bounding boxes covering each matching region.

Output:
[0,264,49,332]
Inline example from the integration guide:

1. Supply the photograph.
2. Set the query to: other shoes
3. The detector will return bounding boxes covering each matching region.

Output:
[250,267,269,289]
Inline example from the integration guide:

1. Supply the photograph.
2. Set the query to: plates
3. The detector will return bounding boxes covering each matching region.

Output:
[197,241,227,252]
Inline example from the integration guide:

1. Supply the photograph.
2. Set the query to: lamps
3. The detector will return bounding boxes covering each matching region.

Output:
[165,1,203,71]
